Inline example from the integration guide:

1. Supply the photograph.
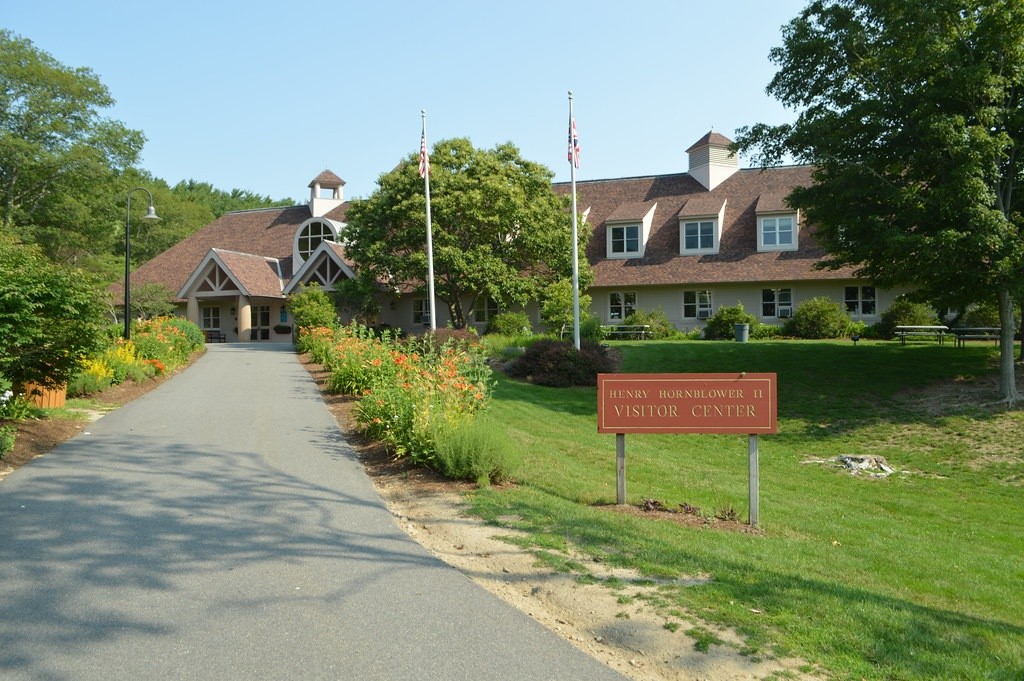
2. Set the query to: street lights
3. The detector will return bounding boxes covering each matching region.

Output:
[125,188,162,340]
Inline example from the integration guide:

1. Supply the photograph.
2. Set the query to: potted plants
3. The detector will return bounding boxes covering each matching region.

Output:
[273,325,292,334]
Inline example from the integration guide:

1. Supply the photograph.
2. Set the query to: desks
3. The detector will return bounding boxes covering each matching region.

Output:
[565,325,650,340]
[897,325,1001,348]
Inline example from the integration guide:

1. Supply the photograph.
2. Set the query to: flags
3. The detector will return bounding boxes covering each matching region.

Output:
[568,114,580,168]
[419,130,430,177]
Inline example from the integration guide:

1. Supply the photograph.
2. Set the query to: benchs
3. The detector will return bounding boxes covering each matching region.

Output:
[561,330,658,341]
[207,330,227,343]
[894,332,1001,351]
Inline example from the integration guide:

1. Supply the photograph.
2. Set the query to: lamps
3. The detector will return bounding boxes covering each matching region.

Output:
[851,336,860,346]
[230,308,235,315]
